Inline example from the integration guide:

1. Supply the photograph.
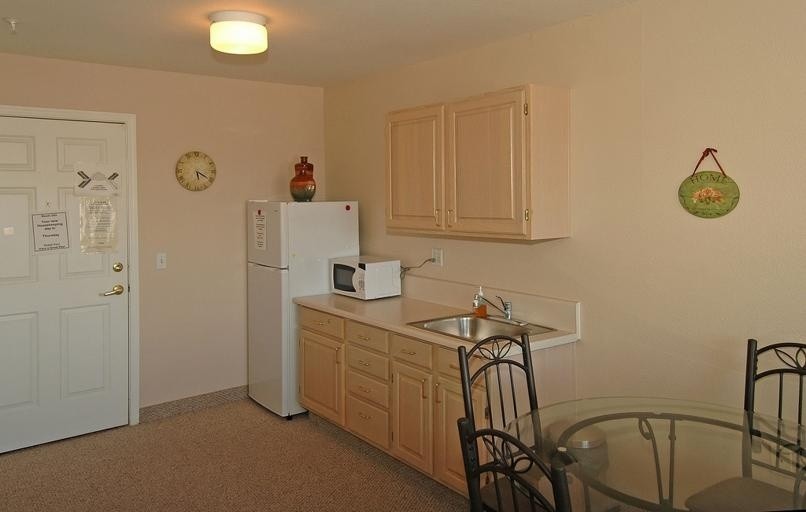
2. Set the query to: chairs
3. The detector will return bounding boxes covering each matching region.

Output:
[684,337,806,512]
[455,414,575,511]
[453,333,592,511]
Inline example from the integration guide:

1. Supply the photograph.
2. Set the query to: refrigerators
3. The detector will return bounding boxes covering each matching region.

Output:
[244,195,359,421]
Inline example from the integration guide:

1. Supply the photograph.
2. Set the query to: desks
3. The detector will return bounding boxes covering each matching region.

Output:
[493,394,806,512]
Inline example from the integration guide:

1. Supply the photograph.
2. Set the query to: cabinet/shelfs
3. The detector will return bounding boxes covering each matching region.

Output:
[382,77,571,247]
[294,303,345,434]
[389,332,578,500]
[342,321,389,456]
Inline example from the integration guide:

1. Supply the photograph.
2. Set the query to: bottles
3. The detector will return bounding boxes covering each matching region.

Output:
[472,294,479,313]
[478,286,485,307]
[294,156,314,178]
[289,167,317,202]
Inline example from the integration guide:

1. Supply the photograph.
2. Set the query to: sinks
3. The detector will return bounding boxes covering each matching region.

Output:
[407,312,556,346]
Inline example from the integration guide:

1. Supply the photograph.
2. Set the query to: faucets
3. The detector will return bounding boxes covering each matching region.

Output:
[472,293,513,320]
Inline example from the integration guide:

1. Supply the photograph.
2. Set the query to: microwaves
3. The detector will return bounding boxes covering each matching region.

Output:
[327,254,403,303]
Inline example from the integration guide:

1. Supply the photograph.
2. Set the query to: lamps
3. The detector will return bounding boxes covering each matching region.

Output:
[206,8,271,58]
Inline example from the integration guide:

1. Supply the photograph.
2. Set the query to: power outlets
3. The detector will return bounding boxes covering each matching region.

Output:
[427,248,444,266]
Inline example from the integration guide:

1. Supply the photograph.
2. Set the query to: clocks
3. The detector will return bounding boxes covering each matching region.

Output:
[173,151,218,192]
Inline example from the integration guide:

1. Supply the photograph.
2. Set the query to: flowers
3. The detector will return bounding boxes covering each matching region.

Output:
[288,156,318,201]
